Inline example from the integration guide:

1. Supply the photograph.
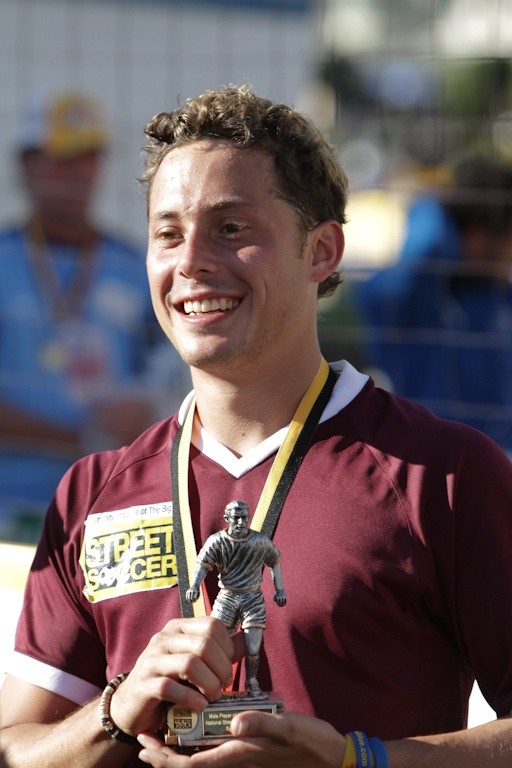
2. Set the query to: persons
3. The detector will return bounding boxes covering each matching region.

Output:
[0,83,512,768]
[350,154,512,460]
[0,94,193,545]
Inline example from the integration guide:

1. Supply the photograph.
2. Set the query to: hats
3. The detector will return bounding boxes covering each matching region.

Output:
[19,93,107,156]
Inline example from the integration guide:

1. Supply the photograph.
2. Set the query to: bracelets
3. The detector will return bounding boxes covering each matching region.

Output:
[349,729,371,768]
[100,671,162,746]
[342,734,357,768]
[368,736,390,768]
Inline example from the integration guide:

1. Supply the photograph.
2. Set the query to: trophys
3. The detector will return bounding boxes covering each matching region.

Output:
[163,500,287,747]
